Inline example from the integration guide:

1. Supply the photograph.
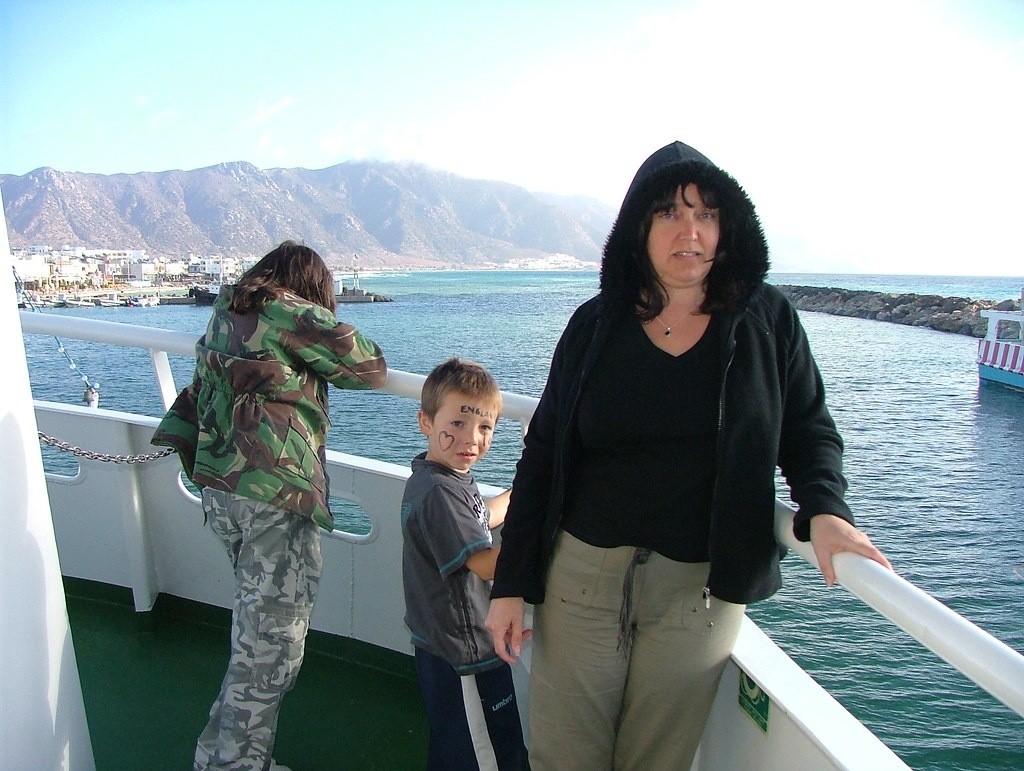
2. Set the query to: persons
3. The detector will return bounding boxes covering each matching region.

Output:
[398,360,534,771]
[482,140,893,771]
[148,238,388,771]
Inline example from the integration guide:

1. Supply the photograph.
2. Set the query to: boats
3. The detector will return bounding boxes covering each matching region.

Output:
[975,287,1024,390]
[16,255,375,309]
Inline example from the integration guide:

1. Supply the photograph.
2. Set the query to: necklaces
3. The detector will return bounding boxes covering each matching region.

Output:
[651,293,706,337]
